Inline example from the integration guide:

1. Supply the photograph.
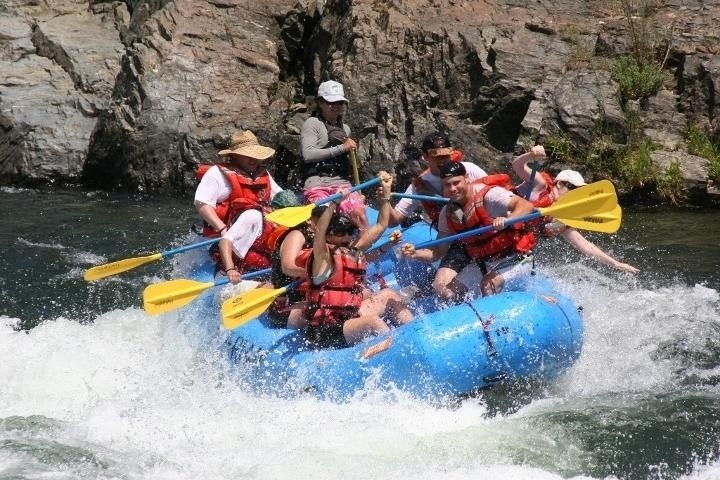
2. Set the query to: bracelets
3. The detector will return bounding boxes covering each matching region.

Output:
[329,199,338,205]
[223,266,239,272]
[217,225,228,235]
[379,245,386,253]
[379,196,393,202]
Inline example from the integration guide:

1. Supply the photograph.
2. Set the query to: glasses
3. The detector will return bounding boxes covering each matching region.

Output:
[326,100,344,106]
[332,221,355,237]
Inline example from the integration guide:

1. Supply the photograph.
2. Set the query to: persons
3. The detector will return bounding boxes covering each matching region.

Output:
[208,188,298,287]
[376,131,489,234]
[193,129,285,238]
[301,79,370,239]
[479,144,642,298]
[305,169,414,349]
[401,160,534,300]
[267,204,336,330]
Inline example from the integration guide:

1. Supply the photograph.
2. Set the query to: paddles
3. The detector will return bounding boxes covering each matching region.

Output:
[405,179,617,250]
[221,235,397,330]
[390,191,623,234]
[83,235,224,281]
[141,267,276,315]
[265,176,383,227]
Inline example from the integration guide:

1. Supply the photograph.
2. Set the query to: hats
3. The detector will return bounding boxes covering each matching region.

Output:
[556,170,588,187]
[438,161,466,178]
[218,130,275,161]
[271,189,299,208]
[420,131,452,150]
[318,82,350,103]
